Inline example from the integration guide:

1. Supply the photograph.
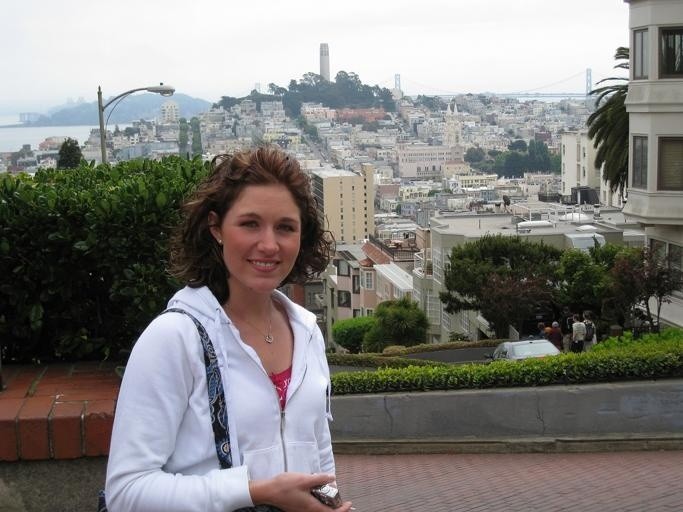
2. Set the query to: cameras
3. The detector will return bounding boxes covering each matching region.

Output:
[310,472,342,509]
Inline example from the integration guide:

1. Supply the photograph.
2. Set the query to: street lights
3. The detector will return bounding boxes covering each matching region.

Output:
[95,80,177,164]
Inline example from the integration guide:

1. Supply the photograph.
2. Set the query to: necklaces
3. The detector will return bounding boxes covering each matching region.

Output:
[223,296,273,343]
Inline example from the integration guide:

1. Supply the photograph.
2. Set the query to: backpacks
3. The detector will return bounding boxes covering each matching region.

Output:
[585,320,594,342]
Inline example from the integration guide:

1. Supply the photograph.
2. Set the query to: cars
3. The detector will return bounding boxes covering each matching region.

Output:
[482,339,564,363]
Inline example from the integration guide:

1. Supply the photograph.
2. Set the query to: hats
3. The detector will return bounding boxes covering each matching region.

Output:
[552,321,559,328]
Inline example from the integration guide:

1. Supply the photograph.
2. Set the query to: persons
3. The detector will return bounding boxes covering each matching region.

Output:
[530,304,598,355]
[103,147,352,511]
